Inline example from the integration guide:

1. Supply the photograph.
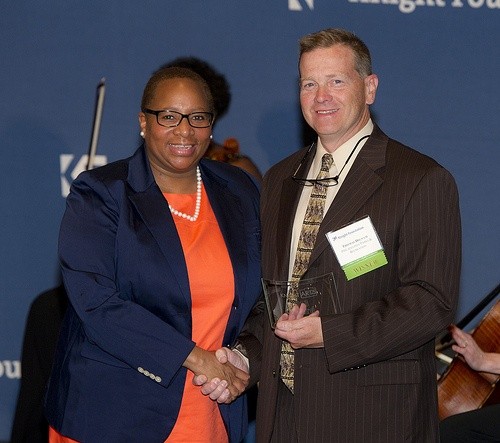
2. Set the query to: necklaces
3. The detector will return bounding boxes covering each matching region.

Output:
[168,165,202,222]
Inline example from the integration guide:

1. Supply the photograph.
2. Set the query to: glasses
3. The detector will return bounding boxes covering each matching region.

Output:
[287,134,371,188]
[142,107,214,128]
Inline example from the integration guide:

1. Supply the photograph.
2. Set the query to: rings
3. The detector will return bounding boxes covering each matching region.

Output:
[463,342,467,348]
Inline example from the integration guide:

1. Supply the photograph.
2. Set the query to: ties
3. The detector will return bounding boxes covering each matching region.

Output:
[280,152,334,395]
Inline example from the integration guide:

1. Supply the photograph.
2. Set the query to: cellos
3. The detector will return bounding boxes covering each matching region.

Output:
[435,299,500,422]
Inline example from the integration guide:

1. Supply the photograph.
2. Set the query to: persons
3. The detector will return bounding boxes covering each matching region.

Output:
[192,29,461,443]
[438,323,500,443]
[44,67,261,443]
